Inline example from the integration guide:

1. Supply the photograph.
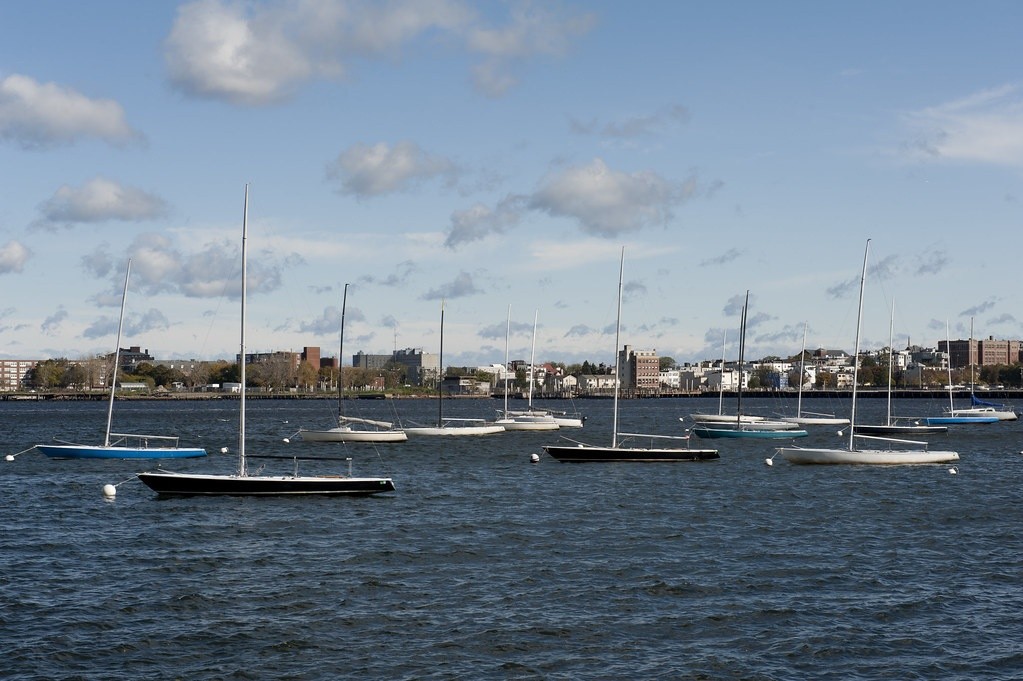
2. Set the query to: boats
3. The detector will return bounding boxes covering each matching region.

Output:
[494,407,588,429]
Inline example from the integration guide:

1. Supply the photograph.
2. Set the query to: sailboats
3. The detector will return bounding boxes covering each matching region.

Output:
[689,306,801,428]
[35,258,208,458]
[540,245,721,463]
[689,288,809,438]
[774,237,961,466]
[493,307,561,431]
[299,284,409,444]
[134,182,398,496]
[919,315,1000,426]
[848,297,949,437]
[941,317,1019,422]
[393,301,506,437]
